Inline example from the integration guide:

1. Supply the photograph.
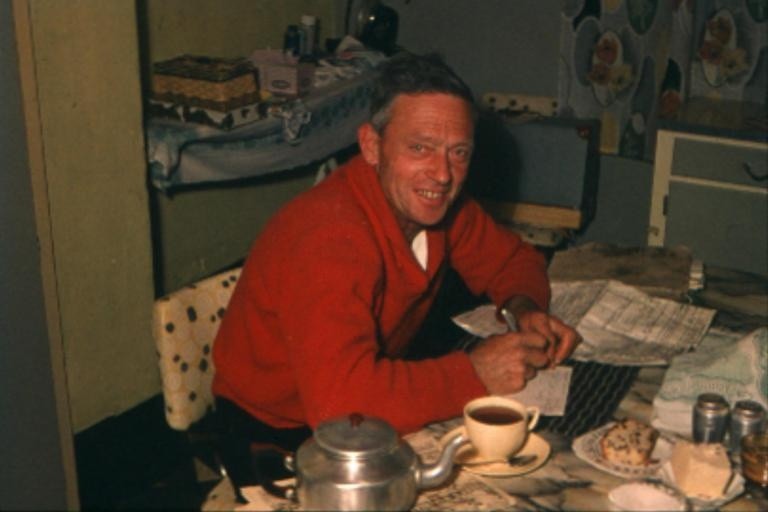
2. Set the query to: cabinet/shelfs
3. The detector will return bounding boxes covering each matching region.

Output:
[649,96,768,276]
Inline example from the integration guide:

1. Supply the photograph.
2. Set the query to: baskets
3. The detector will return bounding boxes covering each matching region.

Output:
[153,53,260,113]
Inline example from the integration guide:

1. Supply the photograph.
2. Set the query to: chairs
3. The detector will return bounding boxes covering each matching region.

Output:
[152,268,241,512]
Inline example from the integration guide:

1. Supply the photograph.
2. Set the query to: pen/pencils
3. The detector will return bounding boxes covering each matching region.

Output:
[500,309,518,332]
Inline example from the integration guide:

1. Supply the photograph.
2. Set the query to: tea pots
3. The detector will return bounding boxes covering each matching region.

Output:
[258,413,471,512]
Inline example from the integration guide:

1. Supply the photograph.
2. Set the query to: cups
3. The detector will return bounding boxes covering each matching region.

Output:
[465,397,540,463]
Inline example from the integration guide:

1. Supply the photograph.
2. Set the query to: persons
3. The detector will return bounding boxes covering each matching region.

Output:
[208,62,585,501]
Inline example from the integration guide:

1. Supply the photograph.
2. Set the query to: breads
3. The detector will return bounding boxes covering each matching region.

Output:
[600,417,658,465]
[670,437,734,498]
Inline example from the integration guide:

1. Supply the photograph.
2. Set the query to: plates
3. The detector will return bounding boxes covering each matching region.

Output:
[439,427,553,478]
[571,420,689,511]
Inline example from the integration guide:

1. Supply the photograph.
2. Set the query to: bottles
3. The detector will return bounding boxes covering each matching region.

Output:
[282,16,318,59]
[693,393,768,458]
